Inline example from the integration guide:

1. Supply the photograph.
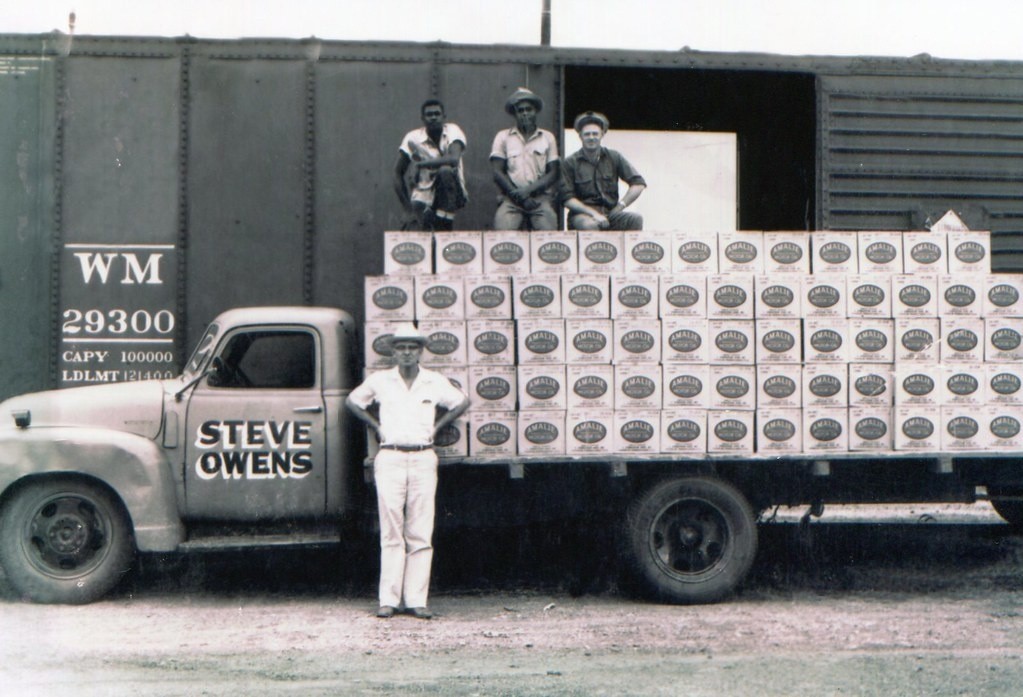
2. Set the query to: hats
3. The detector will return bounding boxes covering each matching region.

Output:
[505,86,543,114]
[574,110,609,135]
[373,322,431,356]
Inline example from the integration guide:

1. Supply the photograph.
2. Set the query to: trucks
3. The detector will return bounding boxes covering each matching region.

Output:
[0,306,1023,607]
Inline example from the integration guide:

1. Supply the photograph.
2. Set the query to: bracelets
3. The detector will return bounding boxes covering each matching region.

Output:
[618,200,626,208]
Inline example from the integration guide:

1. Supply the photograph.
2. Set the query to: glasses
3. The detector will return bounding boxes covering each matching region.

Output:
[393,344,420,351]
[423,111,443,117]
[514,107,537,113]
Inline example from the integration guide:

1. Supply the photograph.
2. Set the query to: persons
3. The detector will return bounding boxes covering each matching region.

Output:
[491,87,561,231]
[559,111,647,233]
[344,326,470,618]
[392,100,469,229]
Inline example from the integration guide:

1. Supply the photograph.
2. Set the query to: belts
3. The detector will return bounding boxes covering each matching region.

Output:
[381,445,433,452]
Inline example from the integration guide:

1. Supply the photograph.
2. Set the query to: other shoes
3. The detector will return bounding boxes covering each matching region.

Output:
[408,212,425,231]
[432,211,455,230]
[377,606,399,617]
[404,608,433,618]
